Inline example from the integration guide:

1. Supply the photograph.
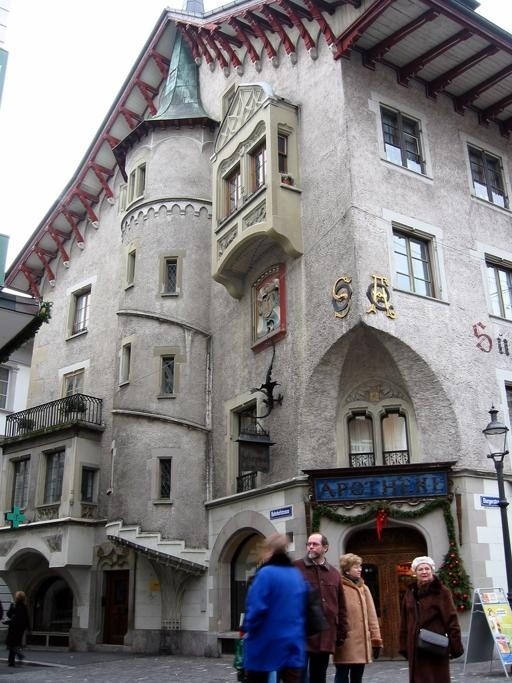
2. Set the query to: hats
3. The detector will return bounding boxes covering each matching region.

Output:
[412,556,435,571]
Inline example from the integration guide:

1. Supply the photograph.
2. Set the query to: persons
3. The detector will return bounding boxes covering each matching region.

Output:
[334,553,383,683]
[0,591,29,667]
[239,532,347,683]
[488,608,512,662]
[398,556,464,683]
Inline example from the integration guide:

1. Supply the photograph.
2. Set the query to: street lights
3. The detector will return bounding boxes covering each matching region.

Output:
[483,400,512,611]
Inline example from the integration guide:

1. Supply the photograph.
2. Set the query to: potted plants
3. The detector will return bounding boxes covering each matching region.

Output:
[16,416,36,435]
[60,396,89,421]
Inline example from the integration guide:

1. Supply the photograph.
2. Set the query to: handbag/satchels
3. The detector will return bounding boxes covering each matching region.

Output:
[303,580,330,638]
[418,628,449,655]
